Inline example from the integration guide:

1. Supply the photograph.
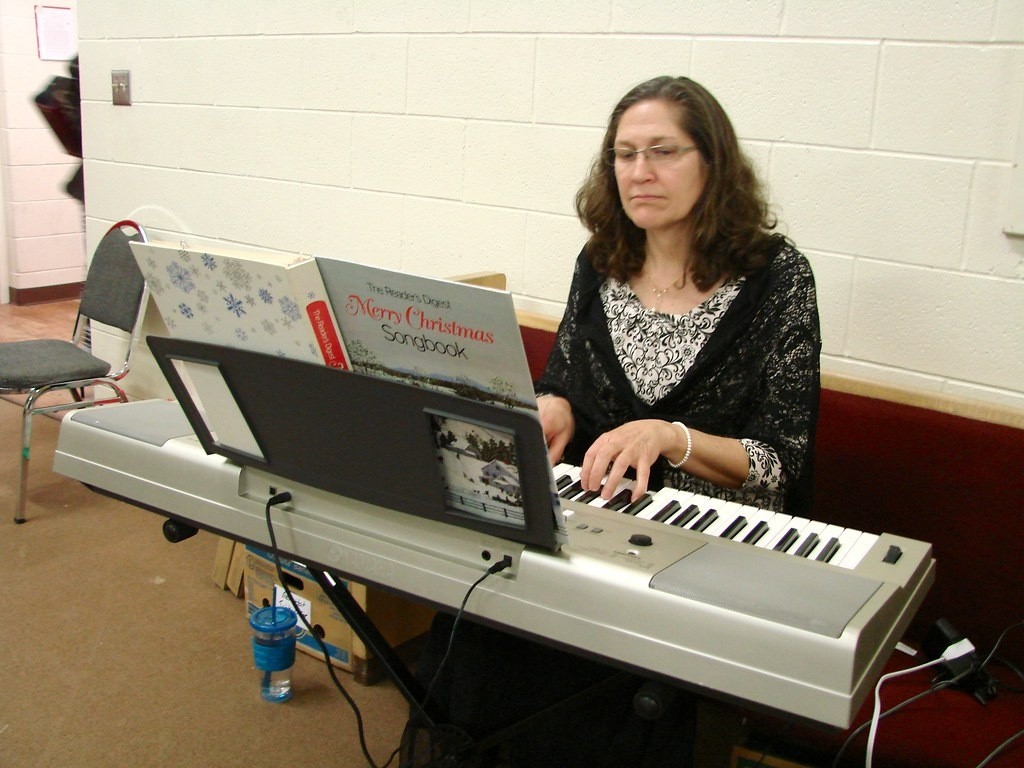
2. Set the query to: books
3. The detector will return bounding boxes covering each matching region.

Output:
[126,239,569,547]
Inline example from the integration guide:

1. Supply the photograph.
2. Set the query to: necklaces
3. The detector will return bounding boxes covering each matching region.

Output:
[642,273,682,312]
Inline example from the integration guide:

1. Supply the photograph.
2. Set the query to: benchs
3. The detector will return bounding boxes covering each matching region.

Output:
[513,310,1024,768]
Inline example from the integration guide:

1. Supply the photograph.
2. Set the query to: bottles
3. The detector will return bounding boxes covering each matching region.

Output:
[250,606,298,702]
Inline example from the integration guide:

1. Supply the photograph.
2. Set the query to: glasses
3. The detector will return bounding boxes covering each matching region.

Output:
[602,143,702,166]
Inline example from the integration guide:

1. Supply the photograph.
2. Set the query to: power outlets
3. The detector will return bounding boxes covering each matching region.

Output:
[112,70,132,105]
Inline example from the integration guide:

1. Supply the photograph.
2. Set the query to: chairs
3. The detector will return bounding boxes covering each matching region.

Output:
[0,218,154,523]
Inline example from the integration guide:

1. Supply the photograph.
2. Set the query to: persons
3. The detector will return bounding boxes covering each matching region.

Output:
[411,77,822,768]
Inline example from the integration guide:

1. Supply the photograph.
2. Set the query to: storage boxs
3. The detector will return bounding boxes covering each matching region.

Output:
[245,542,433,687]
[212,538,234,599]
[227,542,247,599]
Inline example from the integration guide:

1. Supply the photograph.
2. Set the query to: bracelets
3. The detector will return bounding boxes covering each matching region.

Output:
[667,422,691,469]
